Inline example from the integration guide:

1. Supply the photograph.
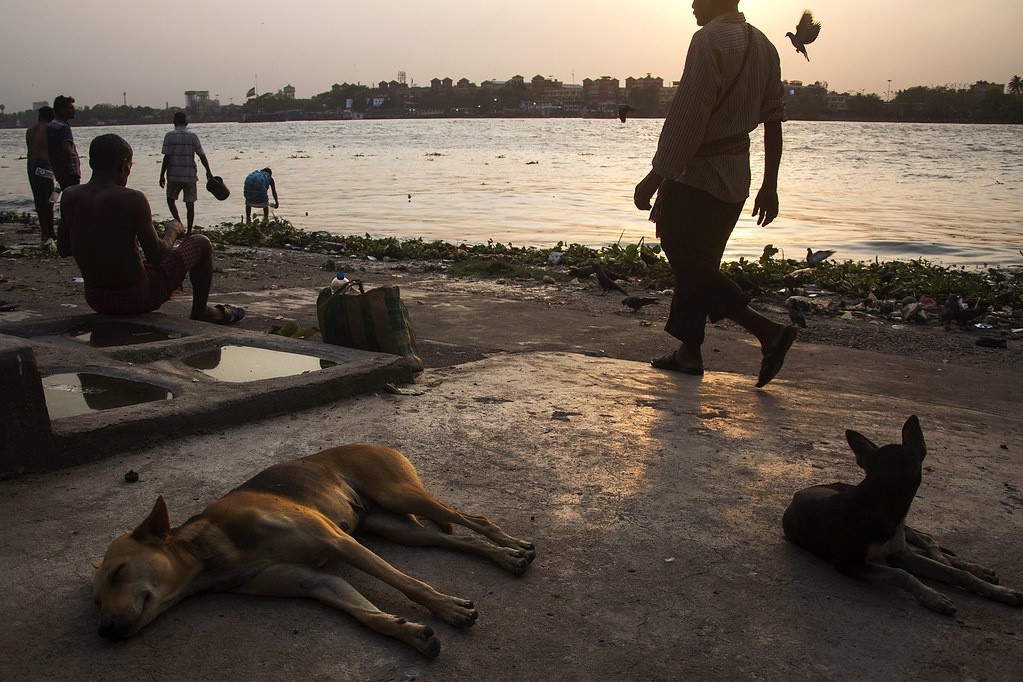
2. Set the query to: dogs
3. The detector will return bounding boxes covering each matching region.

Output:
[90,443,536,662]
[782,414,1023,617]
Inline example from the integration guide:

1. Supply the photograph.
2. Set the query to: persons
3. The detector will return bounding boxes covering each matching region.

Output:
[159,112,214,236]
[633,0,799,387]
[26,106,57,240]
[47,95,81,191]
[56,134,245,325]
[243,168,279,224]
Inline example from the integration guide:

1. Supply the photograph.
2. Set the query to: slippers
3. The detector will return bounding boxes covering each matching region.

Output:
[756,324,797,389]
[214,305,245,324]
[650,350,704,375]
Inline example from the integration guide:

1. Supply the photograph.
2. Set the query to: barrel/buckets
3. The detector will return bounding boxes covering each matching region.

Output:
[206,176,230,201]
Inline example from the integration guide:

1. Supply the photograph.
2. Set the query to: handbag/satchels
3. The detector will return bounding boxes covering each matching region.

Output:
[316,279,424,372]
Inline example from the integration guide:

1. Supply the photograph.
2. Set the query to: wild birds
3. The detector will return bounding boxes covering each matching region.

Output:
[788,298,807,330]
[805,247,838,264]
[938,293,960,329]
[622,296,661,313]
[619,105,640,122]
[785,11,821,63]
[594,265,631,297]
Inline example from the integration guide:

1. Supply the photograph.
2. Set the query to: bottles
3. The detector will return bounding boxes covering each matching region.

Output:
[49,182,61,203]
[331,272,350,294]
